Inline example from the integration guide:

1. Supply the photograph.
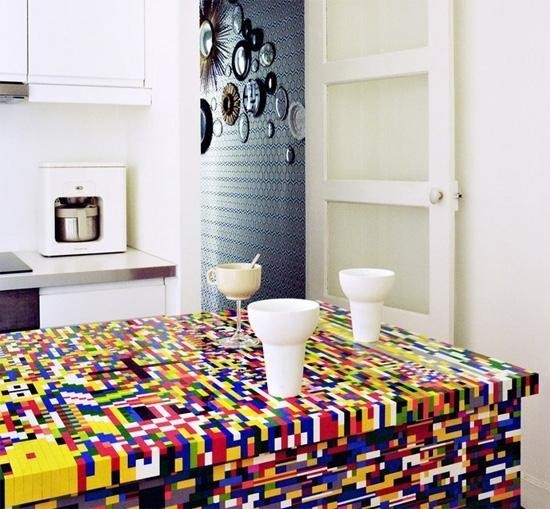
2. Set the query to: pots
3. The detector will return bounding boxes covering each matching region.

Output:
[55,201,98,242]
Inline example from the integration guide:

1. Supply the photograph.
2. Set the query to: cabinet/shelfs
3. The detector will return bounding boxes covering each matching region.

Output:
[0,0,163,107]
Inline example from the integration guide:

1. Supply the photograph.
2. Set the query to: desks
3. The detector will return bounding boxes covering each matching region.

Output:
[2,300,538,509]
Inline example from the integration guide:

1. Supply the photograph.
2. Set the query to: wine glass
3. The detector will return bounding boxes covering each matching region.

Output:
[205,261,262,349]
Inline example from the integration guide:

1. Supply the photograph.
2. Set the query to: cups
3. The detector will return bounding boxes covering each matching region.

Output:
[338,267,395,344]
[247,297,320,398]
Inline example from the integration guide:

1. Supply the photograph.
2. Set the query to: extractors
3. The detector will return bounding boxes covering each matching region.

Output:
[0,82,30,106]
